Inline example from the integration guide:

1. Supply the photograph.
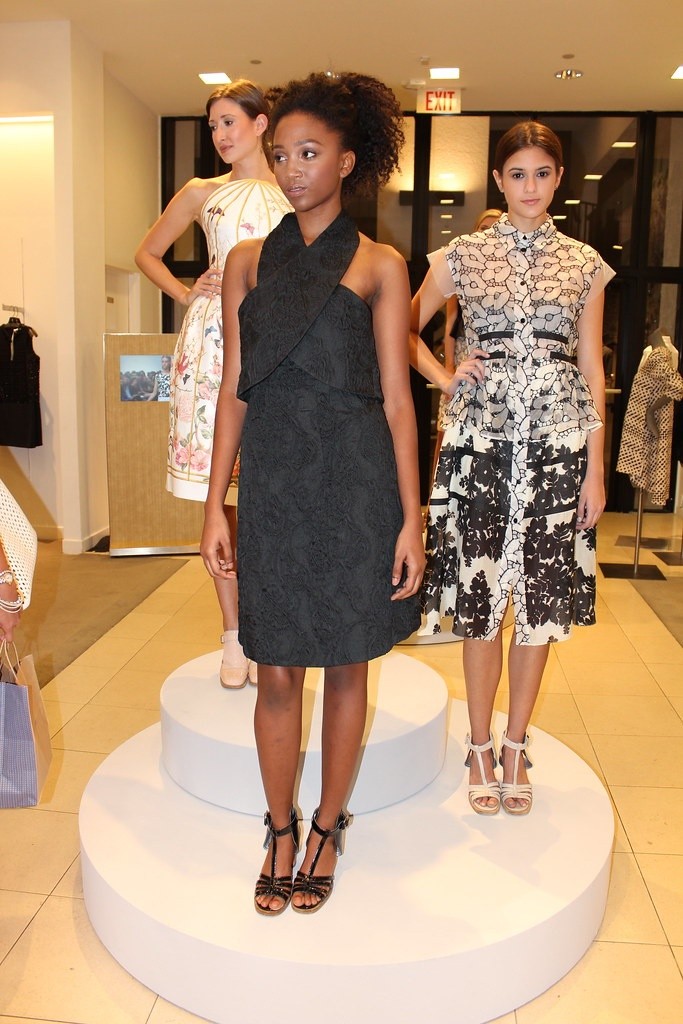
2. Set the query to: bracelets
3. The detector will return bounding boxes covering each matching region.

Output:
[0,569,14,586]
[0,591,22,613]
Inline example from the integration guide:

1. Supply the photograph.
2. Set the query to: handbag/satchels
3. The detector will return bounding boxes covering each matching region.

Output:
[0,639,53,808]
[0,479,38,611]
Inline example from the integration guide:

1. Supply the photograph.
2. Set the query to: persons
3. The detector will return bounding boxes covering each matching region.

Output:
[134,70,426,916]
[409,121,617,817]
[120,356,174,402]
[0,539,23,646]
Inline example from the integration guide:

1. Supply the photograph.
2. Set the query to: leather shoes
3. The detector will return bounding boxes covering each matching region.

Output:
[219,633,259,690]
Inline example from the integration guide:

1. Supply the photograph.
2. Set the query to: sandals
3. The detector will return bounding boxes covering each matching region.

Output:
[498,731,532,816]
[464,729,503,816]
[290,804,354,916]
[252,801,300,916]
